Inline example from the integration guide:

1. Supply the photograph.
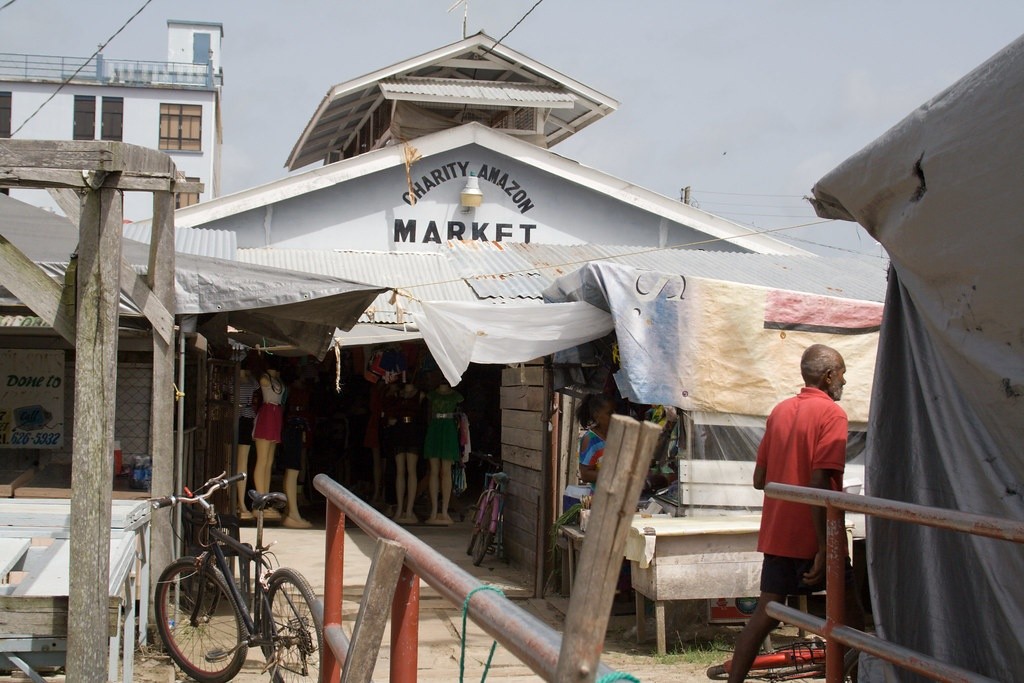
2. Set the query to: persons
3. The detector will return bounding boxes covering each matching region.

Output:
[726,345,865,683]
[235,366,464,529]
[573,392,620,496]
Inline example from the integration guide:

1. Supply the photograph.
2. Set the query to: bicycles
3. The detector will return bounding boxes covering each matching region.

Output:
[146,469,323,683]
[465,450,512,566]
[706,638,858,683]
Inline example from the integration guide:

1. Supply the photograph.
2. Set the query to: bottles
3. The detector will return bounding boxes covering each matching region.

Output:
[130,454,152,489]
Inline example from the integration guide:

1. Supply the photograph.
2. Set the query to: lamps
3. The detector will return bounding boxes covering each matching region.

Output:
[460,172,483,212]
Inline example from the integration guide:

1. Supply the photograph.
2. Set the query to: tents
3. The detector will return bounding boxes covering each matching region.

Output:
[1,191,399,362]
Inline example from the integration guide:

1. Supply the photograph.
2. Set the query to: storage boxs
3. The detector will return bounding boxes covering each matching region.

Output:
[707,598,751,623]
[115,441,122,475]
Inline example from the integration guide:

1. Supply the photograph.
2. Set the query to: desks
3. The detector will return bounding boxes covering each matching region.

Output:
[561,510,853,657]
[0,462,152,683]
